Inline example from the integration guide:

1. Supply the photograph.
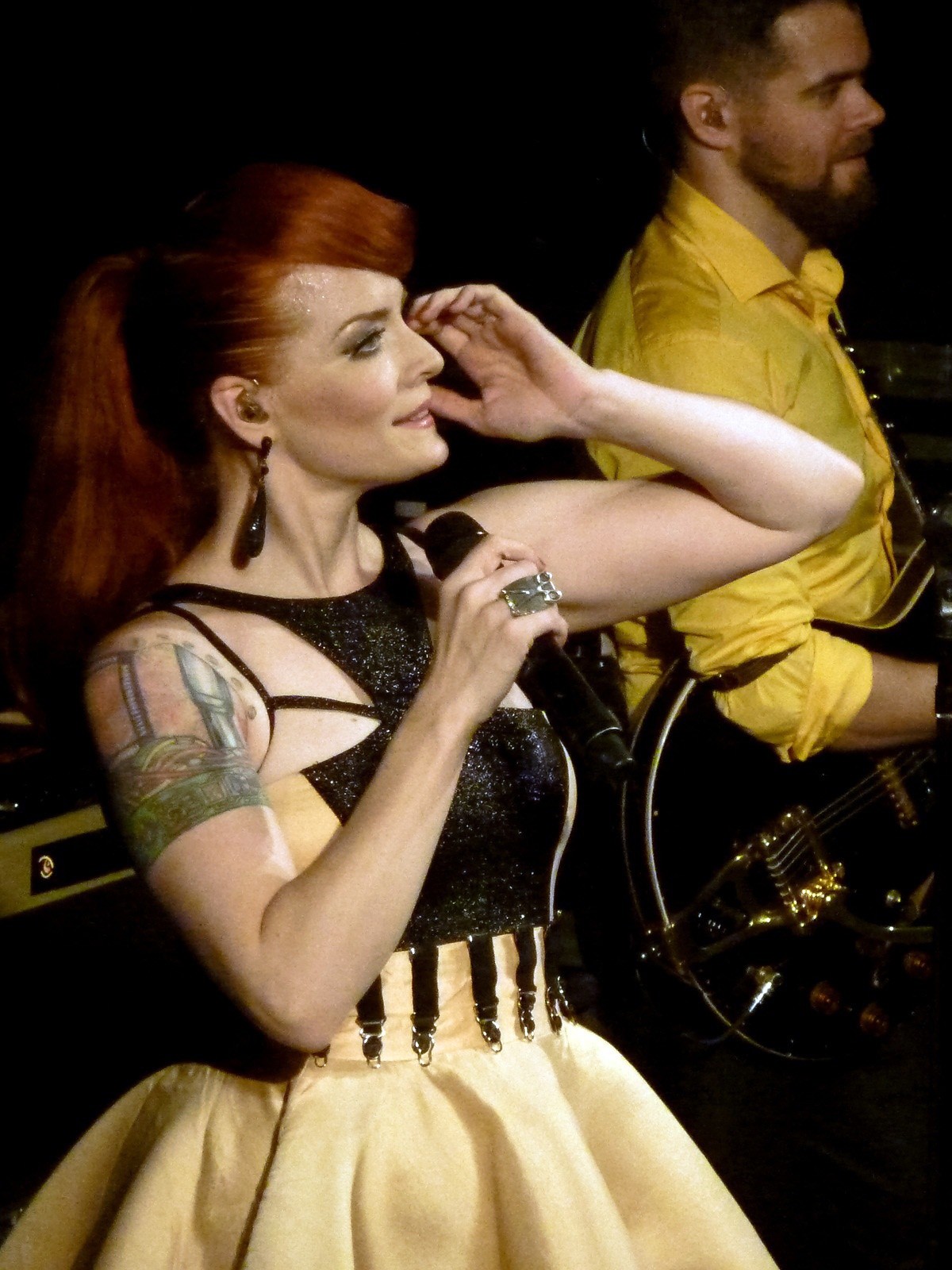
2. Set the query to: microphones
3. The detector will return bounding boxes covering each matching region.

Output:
[422,511,639,784]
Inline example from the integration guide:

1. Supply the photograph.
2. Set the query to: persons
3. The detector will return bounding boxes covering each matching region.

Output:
[582,0,952,767]
[0,169,864,1270]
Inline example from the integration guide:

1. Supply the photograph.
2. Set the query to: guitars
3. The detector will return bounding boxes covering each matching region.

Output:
[619,504,952,1069]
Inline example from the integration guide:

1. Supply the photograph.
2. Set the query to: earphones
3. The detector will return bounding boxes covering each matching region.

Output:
[235,390,270,425]
[696,98,728,133]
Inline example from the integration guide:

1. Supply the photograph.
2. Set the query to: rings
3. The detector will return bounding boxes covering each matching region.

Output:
[500,571,563,617]
